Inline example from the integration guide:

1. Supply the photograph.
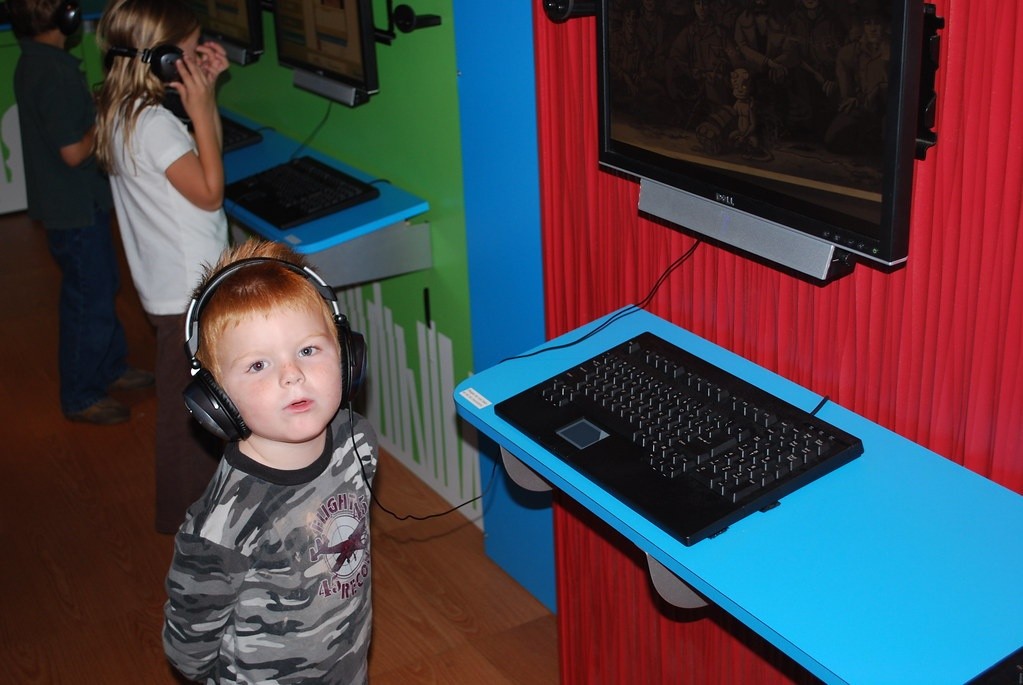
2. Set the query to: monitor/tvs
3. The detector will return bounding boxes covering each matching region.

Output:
[595,0,924,276]
[272,0,379,95]
[206,0,264,55]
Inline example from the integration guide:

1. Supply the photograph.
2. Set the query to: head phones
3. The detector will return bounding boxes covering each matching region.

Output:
[107,44,191,85]
[183,256,367,442]
[56,0,82,37]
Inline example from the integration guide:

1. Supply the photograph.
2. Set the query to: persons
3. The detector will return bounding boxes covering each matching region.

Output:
[162,237,380,685]
[86,0,232,534]
[1,0,157,426]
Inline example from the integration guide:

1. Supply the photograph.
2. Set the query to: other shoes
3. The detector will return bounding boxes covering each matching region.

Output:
[103,365,154,393]
[69,396,131,425]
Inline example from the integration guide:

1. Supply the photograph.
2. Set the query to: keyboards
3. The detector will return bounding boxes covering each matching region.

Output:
[225,156,380,231]
[493,332,863,548]
